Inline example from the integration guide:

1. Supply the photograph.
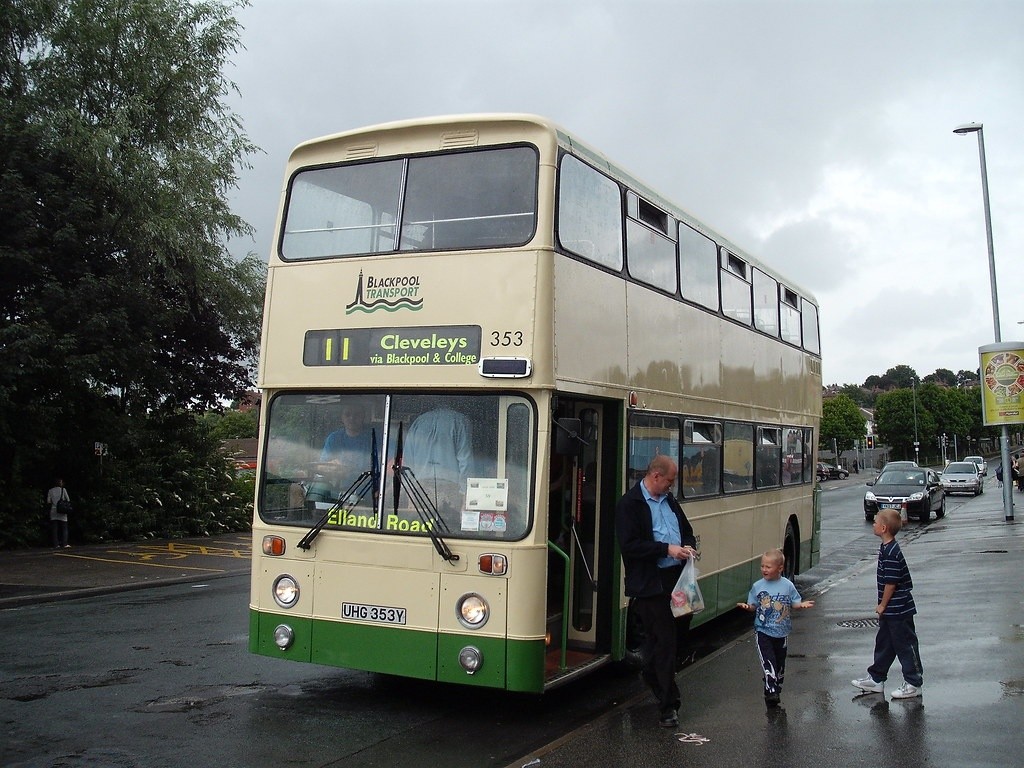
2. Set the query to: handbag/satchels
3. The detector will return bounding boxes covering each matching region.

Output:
[670,550,705,618]
[57,498,72,514]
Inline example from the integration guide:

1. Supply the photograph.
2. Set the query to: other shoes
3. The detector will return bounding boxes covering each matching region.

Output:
[56,545,62,548]
[64,545,71,548]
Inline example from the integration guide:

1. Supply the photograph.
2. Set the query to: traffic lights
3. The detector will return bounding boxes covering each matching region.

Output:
[866,436,874,449]
[944,436,948,446]
[936,436,940,448]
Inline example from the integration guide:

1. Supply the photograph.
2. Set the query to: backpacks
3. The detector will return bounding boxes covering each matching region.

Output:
[995,465,1003,481]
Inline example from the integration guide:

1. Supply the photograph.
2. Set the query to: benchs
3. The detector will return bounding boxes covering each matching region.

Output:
[560,239,801,346]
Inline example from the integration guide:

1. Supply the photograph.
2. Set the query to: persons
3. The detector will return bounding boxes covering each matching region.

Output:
[401,400,473,534]
[853,458,858,474]
[995,462,1003,489]
[737,549,816,704]
[48,479,72,549]
[318,404,403,506]
[1010,450,1024,493]
[616,454,698,727]
[851,509,923,698]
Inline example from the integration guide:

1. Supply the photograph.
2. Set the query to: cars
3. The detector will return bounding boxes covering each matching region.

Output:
[876,462,919,473]
[864,466,946,522]
[963,456,988,476]
[815,462,849,481]
[937,462,984,496]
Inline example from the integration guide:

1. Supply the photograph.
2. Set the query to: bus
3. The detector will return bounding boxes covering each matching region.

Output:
[249,114,822,693]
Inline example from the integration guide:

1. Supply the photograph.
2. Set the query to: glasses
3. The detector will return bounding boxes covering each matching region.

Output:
[659,474,675,485]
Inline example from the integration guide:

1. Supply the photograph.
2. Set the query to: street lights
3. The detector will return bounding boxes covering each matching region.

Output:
[953,121,1014,521]
[910,376,919,464]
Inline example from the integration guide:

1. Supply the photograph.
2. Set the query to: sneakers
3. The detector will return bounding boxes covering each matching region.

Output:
[659,710,679,728]
[851,674,884,692]
[765,693,781,706]
[890,681,922,699]
